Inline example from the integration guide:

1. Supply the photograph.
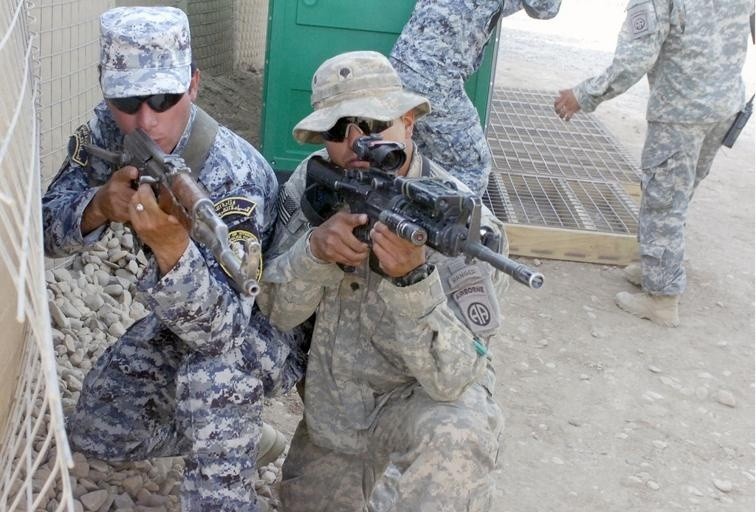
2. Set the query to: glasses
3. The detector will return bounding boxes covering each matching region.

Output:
[318,117,393,143]
[109,94,185,115]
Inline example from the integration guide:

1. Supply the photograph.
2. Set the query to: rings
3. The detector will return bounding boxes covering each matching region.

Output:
[135,203,144,212]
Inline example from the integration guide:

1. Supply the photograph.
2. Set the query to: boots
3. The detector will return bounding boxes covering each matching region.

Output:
[256,422,288,469]
[615,263,679,328]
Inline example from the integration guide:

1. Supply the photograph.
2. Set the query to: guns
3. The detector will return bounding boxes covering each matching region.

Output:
[85,127,260,298]
[300,132,544,290]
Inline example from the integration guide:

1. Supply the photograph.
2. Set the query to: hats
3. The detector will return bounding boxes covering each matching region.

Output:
[98,6,192,99]
[293,50,431,147]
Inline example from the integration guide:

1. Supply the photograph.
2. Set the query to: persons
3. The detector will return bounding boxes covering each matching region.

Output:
[255,51,509,512]
[41,5,308,512]
[554,0,756,328]
[389,0,562,201]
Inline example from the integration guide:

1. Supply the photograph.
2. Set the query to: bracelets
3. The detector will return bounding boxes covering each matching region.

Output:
[397,264,429,287]
